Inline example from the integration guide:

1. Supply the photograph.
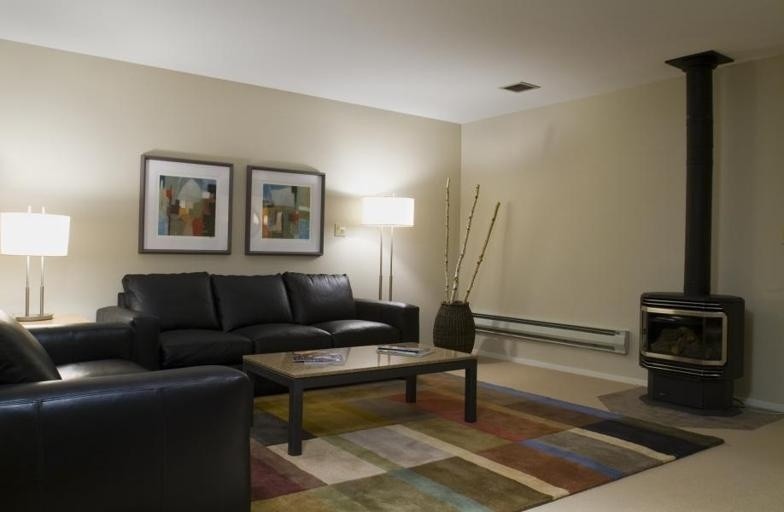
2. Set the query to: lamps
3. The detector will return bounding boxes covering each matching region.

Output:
[357,193,415,303]
[0,202,74,323]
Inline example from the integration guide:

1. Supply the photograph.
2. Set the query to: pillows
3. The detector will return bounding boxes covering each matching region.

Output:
[280,270,357,324]
[204,269,291,331]
[120,268,216,332]
[0,319,61,384]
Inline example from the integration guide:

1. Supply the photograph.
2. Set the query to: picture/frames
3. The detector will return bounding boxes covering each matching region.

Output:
[136,151,235,256]
[243,162,327,258]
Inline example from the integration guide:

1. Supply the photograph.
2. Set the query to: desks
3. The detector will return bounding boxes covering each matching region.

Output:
[12,311,94,332]
[237,339,484,458]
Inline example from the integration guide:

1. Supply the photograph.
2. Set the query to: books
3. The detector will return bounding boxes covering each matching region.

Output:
[375,341,434,357]
[280,348,342,365]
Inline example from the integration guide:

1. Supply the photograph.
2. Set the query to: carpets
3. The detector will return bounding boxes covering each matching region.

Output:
[251,371,729,512]
[596,382,782,431]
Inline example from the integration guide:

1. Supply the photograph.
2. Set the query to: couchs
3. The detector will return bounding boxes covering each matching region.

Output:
[97,270,421,401]
[0,316,259,512]
